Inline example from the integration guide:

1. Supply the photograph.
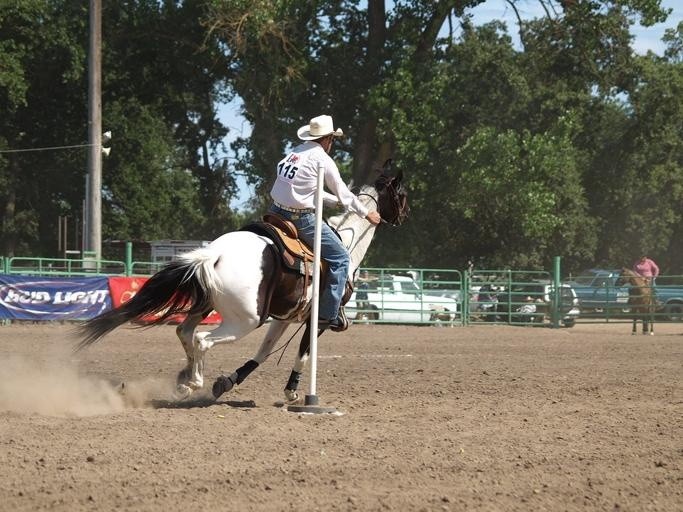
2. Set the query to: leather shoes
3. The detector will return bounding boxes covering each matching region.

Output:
[317,317,341,329]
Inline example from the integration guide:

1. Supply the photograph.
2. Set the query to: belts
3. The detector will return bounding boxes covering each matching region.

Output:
[273,201,313,214]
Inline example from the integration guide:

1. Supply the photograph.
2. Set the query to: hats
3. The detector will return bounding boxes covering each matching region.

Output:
[296,114,343,140]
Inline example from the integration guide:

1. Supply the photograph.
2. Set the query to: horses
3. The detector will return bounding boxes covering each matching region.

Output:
[614,266,656,336]
[62,158,412,405]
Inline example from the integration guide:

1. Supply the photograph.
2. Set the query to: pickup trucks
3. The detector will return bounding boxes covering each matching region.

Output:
[562,268,683,322]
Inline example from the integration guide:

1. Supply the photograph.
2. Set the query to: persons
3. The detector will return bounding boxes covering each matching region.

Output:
[625,251,660,306]
[265,115,382,332]
[351,270,551,325]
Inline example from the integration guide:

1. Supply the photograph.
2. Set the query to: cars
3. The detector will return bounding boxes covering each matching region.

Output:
[340,268,579,328]
